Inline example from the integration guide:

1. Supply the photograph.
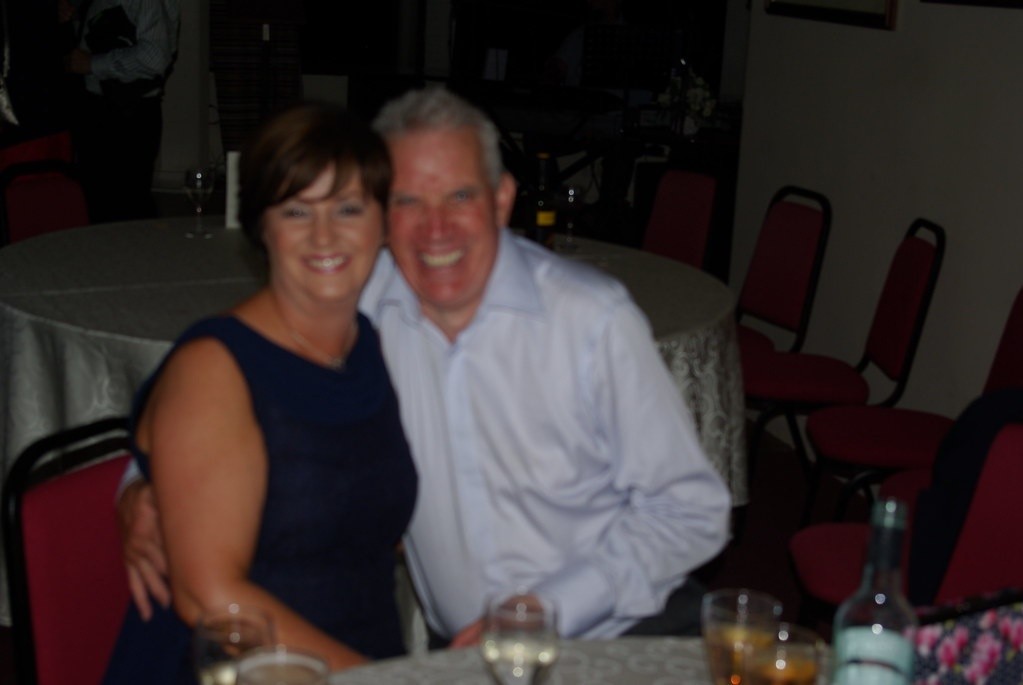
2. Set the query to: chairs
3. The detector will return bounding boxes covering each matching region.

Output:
[742,216,947,507]
[732,182,835,374]
[641,161,722,269]
[780,276,1023,645]
[1,408,162,684]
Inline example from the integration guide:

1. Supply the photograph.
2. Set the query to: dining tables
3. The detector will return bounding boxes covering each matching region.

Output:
[3,208,750,536]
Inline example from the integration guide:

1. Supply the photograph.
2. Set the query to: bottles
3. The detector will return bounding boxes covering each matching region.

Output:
[526,147,555,251]
[700,587,825,682]
[833,496,922,683]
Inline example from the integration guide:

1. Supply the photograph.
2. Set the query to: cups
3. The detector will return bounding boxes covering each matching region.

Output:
[484,591,558,685]
[197,603,331,685]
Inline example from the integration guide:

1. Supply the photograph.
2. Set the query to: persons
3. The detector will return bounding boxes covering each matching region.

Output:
[57,1,182,224]
[115,86,734,654]
[94,104,419,685]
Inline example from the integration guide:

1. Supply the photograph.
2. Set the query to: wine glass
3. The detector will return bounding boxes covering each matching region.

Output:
[558,186,581,251]
[178,169,214,240]
